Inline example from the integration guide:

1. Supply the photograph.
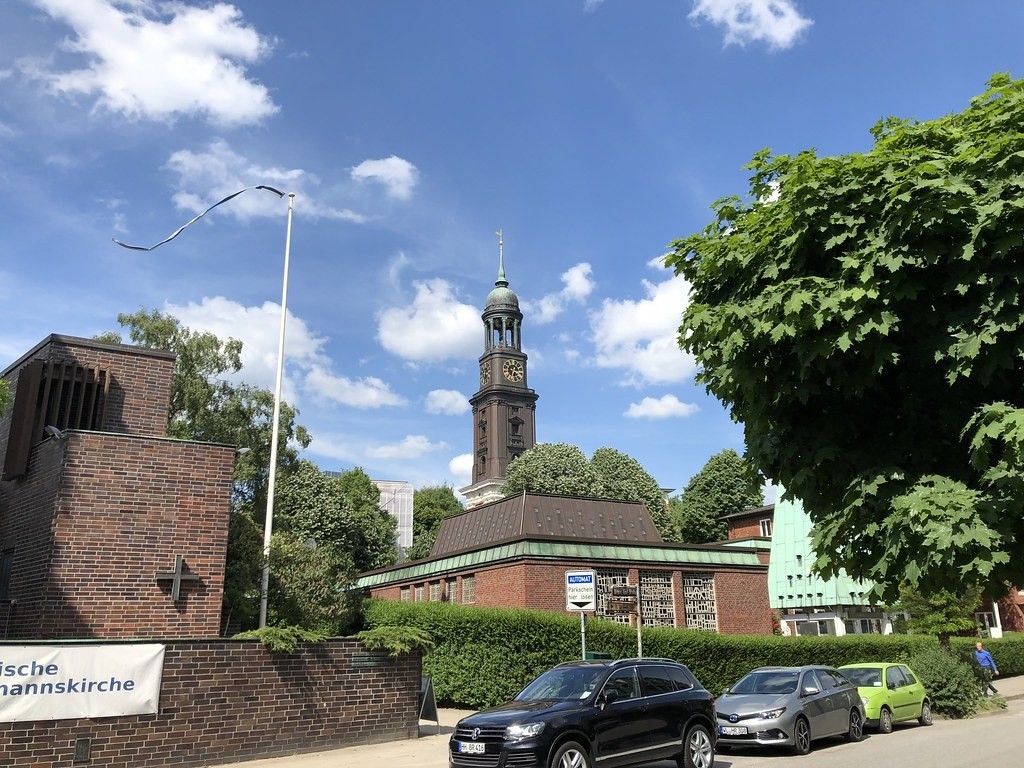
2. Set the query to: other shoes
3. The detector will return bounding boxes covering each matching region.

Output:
[993,691,999,696]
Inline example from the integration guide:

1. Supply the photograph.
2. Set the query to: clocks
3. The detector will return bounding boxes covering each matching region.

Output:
[503,359,524,383]
[480,361,491,386]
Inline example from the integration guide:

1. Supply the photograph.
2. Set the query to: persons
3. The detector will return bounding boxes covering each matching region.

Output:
[975,642,1000,693]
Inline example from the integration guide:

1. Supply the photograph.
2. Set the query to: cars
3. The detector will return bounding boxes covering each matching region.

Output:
[836,662,932,734]
[711,665,866,755]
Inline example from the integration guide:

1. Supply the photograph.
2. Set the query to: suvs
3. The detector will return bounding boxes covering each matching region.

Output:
[447,657,718,768]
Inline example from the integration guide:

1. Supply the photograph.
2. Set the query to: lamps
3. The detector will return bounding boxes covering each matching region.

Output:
[237,447,251,458]
[44,423,65,442]
